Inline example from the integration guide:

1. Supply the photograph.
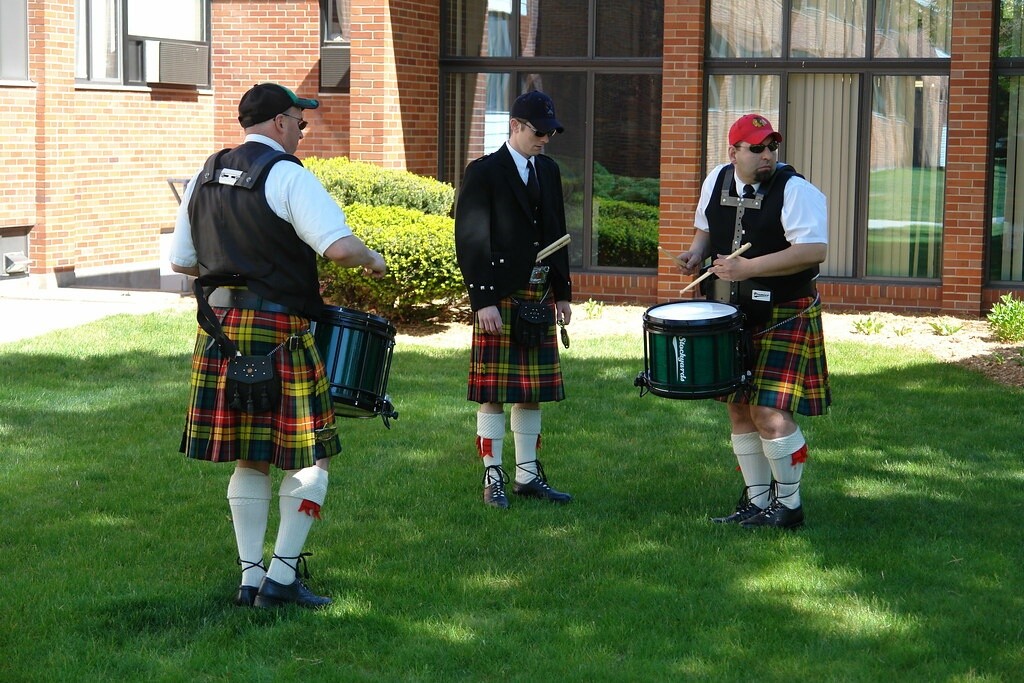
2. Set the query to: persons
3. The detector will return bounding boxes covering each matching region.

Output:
[675,114,831,529]
[456,91,573,511]
[172,82,387,610]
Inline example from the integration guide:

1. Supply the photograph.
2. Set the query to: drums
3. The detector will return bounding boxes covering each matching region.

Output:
[306,304,397,420]
[633,299,754,401]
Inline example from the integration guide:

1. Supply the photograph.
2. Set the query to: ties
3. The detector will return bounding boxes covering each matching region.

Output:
[526,160,540,205]
[743,184,756,199]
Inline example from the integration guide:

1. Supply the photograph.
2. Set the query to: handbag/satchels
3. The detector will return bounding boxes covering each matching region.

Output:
[699,265,710,296]
[512,303,554,348]
[222,355,280,416]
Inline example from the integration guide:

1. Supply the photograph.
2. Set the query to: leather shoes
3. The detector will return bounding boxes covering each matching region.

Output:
[254,553,333,610]
[738,480,805,530]
[710,484,773,524]
[512,459,572,505]
[237,557,267,609]
[482,465,509,511]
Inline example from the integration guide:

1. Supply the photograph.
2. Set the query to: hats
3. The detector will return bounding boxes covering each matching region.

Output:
[237,83,319,128]
[510,89,565,134]
[729,114,783,146]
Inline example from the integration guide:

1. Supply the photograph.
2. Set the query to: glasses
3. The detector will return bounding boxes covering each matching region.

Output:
[273,114,308,131]
[517,120,557,138]
[735,138,780,153]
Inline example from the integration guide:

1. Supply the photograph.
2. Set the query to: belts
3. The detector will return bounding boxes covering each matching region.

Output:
[201,287,300,315]
